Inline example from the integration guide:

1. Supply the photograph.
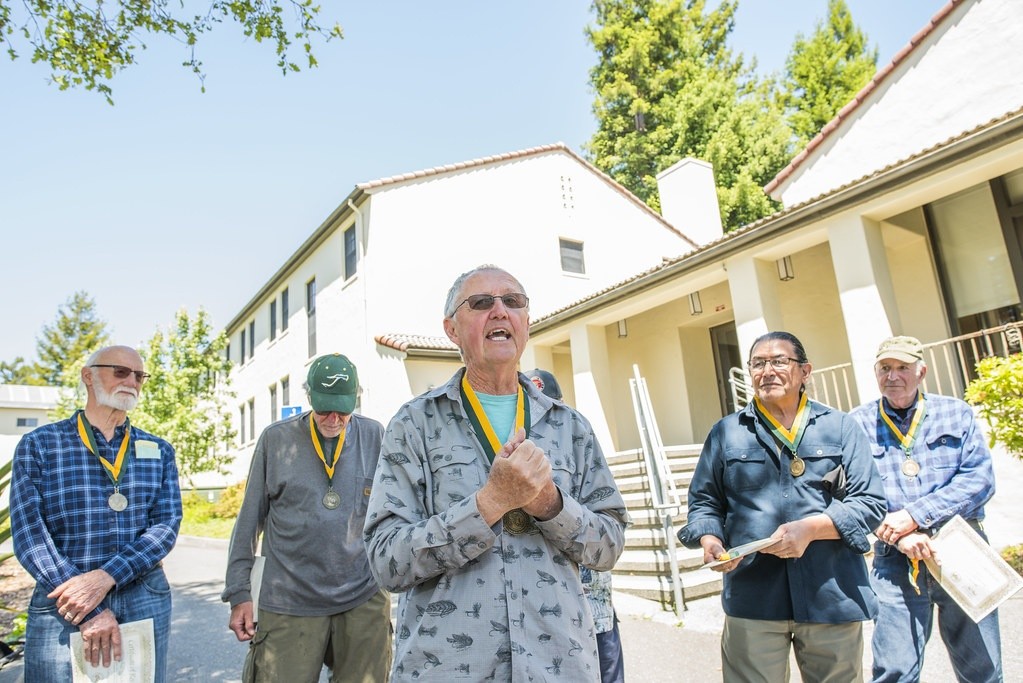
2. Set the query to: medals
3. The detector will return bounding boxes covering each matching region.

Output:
[108,493,128,512]
[503,509,530,536]
[323,492,341,509]
[903,460,920,476]
[790,458,805,477]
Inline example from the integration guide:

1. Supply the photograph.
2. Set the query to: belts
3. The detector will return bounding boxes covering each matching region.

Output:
[920,518,981,536]
[156,562,163,570]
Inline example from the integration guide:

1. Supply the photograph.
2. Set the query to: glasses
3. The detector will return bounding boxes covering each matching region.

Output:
[91,364,150,384]
[747,357,803,371]
[450,293,529,319]
[315,411,348,416]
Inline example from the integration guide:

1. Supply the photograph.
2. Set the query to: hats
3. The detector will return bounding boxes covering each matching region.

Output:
[875,336,924,363]
[523,368,562,398]
[307,353,358,414]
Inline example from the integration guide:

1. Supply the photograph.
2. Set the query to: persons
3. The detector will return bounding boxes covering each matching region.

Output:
[678,332,887,683]
[219,353,394,683]
[523,368,625,682]
[848,336,1004,683]
[9,346,183,683]
[364,266,629,683]
